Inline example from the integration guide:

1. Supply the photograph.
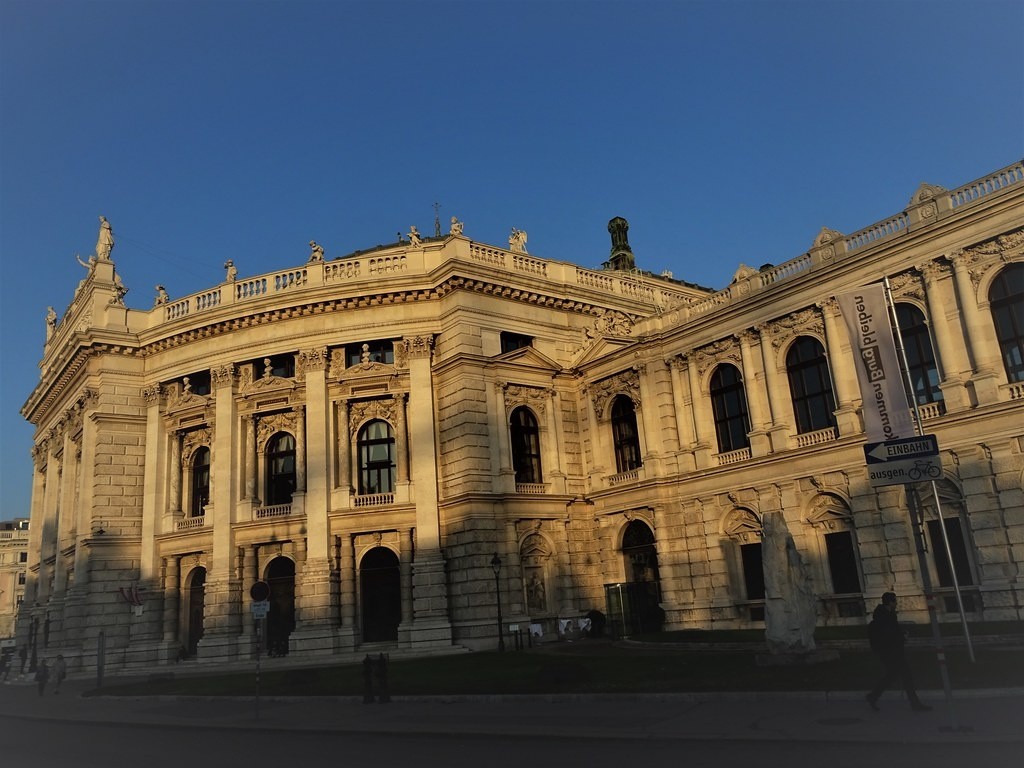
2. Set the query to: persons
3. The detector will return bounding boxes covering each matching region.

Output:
[308,240,323,261]
[512,227,521,241]
[168,344,406,459]
[864,593,932,712]
[268,640,288,658]
[406,226,422,248]
[45,305,58,327]
[223,258,238,282]
[0,644,67,697]
[449,216,463,236]
[72,215,126,292]
[530,571,545,609]
[154,285,169,305]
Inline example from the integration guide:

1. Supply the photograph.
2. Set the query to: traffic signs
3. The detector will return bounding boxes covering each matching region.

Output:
[862,435,945,489]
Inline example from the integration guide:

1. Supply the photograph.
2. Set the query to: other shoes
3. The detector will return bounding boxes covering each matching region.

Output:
[866,693,880,710]
[912,700,934,712]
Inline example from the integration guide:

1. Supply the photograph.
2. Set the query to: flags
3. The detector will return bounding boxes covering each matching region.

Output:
[120,587,144,606]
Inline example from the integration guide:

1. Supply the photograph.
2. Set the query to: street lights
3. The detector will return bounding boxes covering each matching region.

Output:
[489,550,506,652]
